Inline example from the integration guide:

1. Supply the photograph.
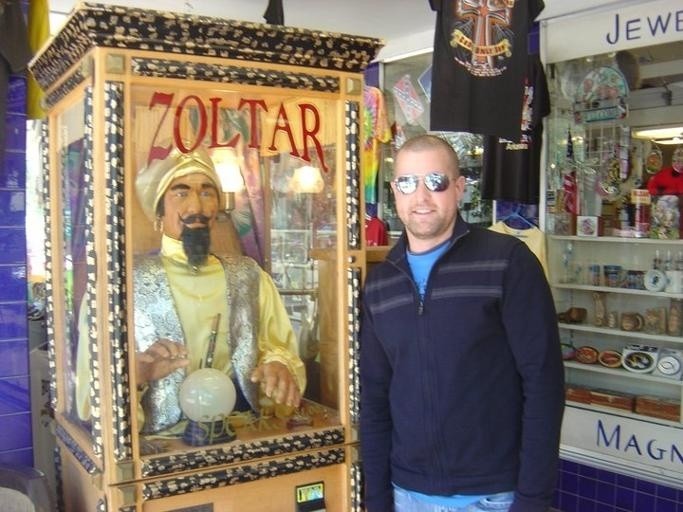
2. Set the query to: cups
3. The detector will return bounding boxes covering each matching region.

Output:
[665,270,683,294]
[620,313,643,332]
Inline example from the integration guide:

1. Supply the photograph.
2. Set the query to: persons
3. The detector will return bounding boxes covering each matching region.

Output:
[646,147,683,197]
[355,135,569,512]
[73,139,310,435]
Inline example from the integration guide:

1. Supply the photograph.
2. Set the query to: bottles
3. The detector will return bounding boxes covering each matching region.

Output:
[559,242,642,291]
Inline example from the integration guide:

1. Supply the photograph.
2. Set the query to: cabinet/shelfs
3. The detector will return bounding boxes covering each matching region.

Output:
[376,0,682,491]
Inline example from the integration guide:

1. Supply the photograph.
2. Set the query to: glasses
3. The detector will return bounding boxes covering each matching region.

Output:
[394,172,456,195]
